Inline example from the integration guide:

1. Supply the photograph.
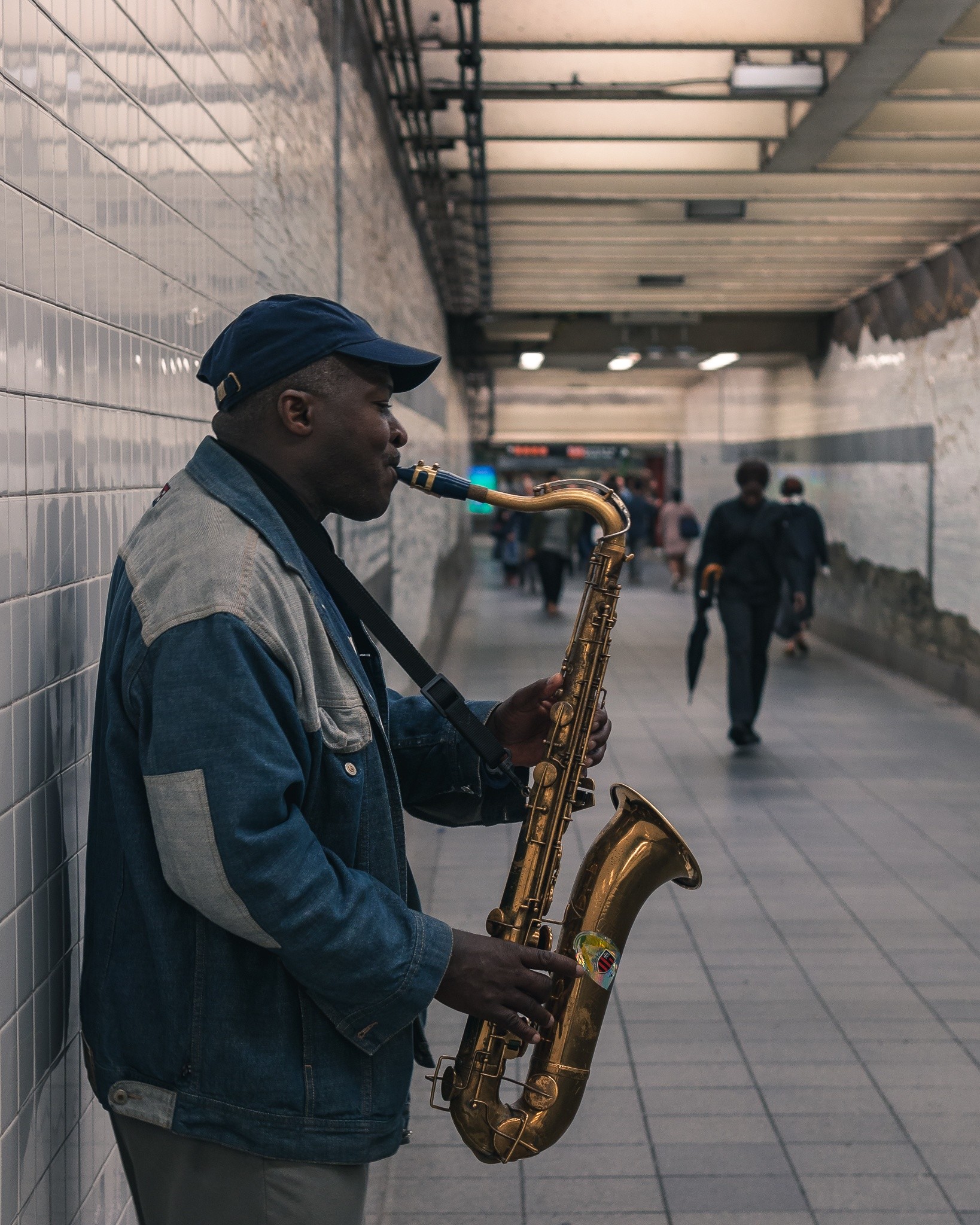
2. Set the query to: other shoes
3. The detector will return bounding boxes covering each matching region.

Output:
[729,723,761,744]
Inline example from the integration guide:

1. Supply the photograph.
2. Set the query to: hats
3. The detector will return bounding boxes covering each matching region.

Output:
[195,293,444,415]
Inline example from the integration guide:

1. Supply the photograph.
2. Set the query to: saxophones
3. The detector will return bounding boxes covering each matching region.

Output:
[394,460,702,1164]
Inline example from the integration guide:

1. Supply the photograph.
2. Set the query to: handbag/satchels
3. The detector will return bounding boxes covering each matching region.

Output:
[680,515,700,539]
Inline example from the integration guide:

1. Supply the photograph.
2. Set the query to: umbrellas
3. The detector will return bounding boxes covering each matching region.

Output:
[687,562,723,705]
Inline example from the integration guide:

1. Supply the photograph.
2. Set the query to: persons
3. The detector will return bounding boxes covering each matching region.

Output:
[490,467,699,616]
[775,476,833,656]
[693,459,806,747]
[78,293,611,1225]
